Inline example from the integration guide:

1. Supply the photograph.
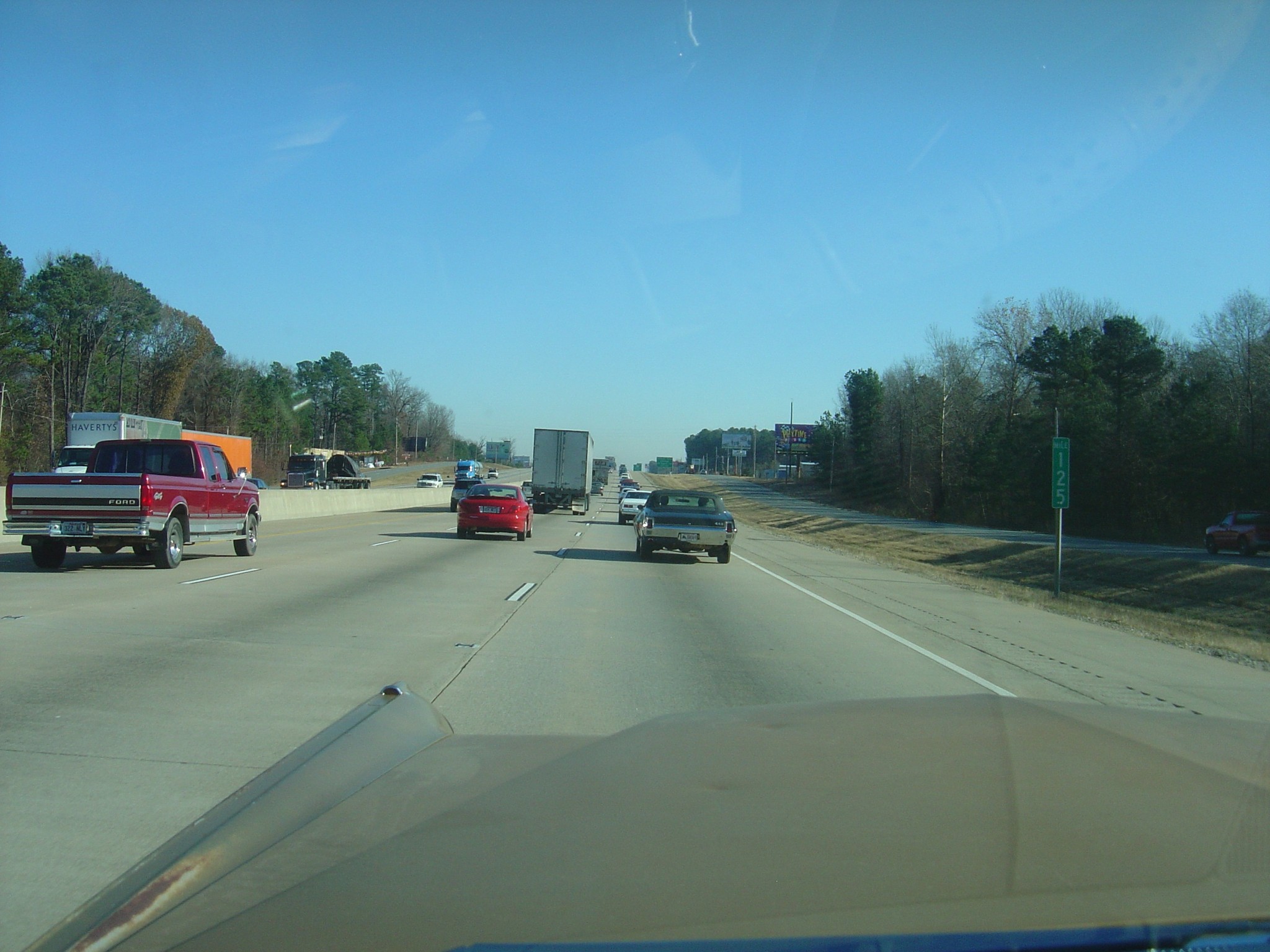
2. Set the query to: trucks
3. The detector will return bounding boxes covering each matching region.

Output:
[454,459,484,481]
[591,456,617,486]
[531,428,596,516]
[47,411,183,474]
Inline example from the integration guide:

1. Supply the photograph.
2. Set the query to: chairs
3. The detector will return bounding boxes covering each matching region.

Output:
[159,449,194,476]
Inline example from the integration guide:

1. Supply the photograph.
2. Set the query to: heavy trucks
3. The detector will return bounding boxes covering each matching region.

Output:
[279,454,372,490]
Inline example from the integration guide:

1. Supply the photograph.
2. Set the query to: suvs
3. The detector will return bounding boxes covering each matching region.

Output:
[450,477,494,513]
[487,468,499,479]
[1204,509,1270,558]
[416,473,443,488]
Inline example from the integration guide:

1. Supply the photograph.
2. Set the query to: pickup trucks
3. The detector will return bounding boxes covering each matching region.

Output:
[1,438,262,569]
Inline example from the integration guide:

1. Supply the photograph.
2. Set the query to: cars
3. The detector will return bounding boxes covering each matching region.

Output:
[456,484,537,541]
[686,468,695,474]
[245,478,271,489]
[618,490,661,525]
[618,488,640,504]
[633,488,737,564]
[700,469,707,475]
[520,480,534,498]
[618,464,642,491]
[590,482,605,496]
